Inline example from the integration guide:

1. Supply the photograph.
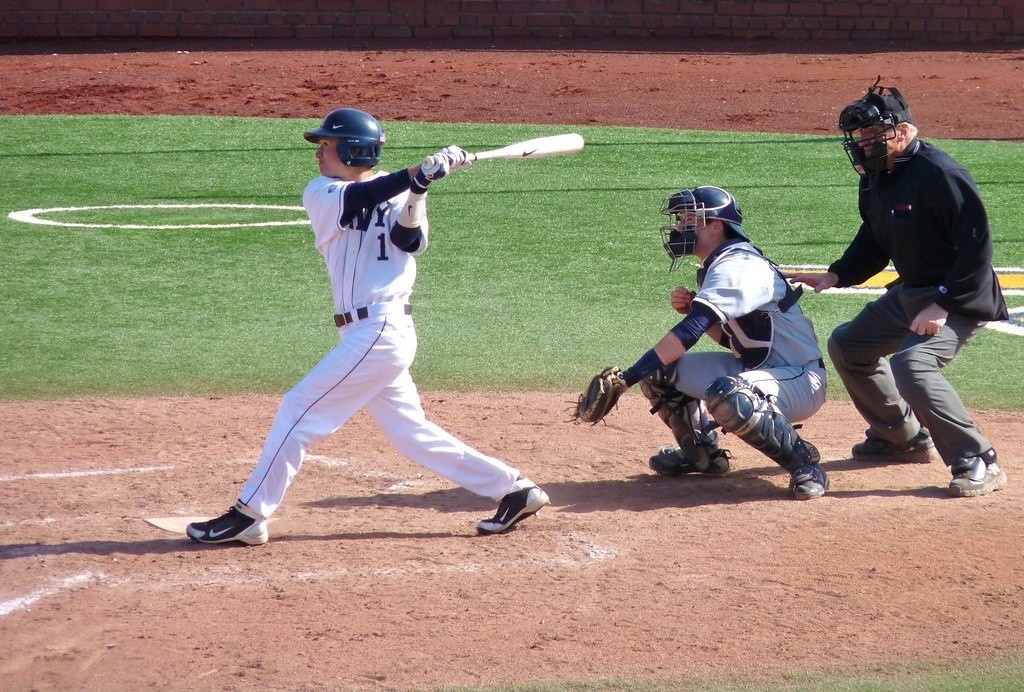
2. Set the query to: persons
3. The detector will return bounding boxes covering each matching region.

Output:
[784,75,1008,498]
[186,108,549,543]
[564,186,829,500]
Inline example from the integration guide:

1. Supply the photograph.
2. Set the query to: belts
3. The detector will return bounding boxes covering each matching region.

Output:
[334,304,413,328]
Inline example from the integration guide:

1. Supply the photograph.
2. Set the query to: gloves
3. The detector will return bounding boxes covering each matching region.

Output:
[431,145,471,172]
[410,156,449,194]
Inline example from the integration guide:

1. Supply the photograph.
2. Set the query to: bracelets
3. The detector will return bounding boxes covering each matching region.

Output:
[410,177,428,194]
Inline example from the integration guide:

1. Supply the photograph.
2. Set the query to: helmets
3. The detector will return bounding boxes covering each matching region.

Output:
[304,108,385,166]
[669,186,750,242]
[839,93,912,132]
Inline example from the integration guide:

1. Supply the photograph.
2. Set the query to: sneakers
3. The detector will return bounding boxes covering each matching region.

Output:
[790,462,830,499]
[476,478,549,533]
[949,448,1008,497]
[852,424,938,462]
[186,505,268,545]
[649,446,732,475]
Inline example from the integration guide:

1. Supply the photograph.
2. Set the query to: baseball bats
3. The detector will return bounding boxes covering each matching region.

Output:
[422,133,584,169]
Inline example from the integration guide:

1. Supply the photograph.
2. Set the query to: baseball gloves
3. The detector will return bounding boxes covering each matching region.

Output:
[562,365,622,427]
